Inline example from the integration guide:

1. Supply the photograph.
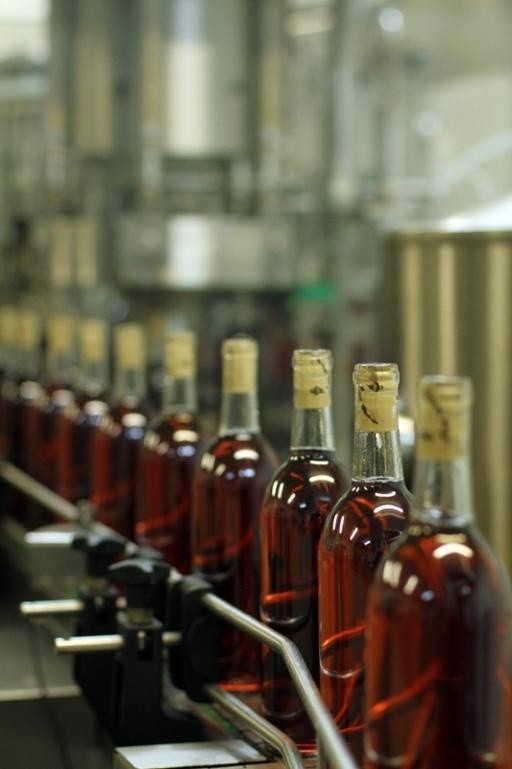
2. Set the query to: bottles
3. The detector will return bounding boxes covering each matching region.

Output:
[136,331,212,573]
[318,359,416,765]
[191,336,279,691]
[260,347,358,737]
[365,375,512,768]
[1,307,109,529]
[92,325,152,537]
